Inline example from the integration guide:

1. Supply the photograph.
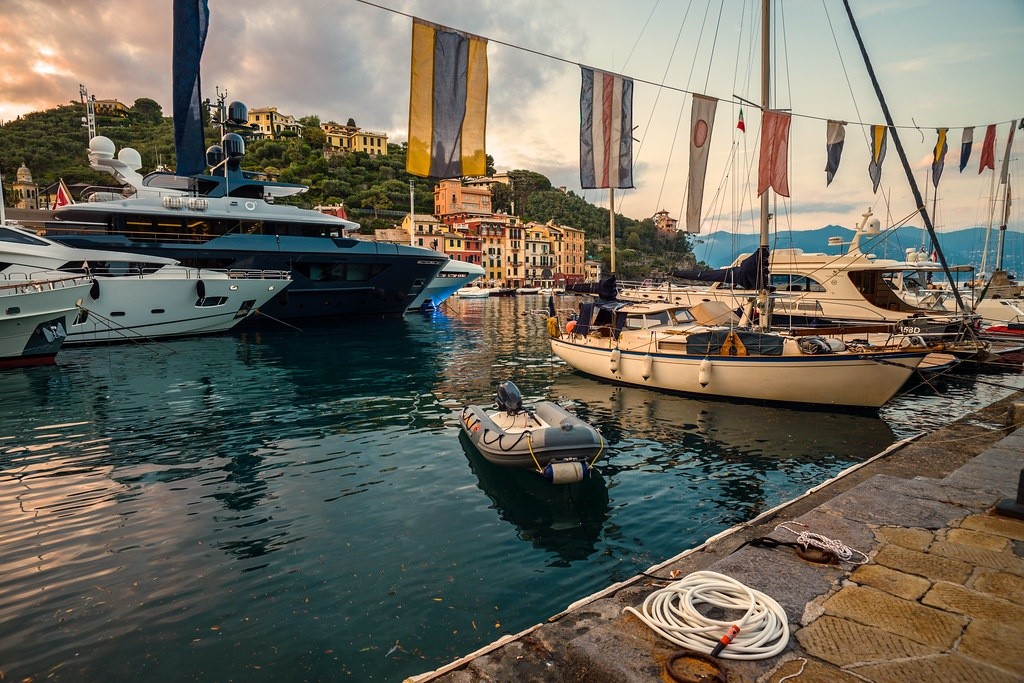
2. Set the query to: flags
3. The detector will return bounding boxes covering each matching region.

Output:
[174,1,211,174]
[929,250,938,262]
[580,63,635,190]
[52,184,71,210]
[405,18,488,178]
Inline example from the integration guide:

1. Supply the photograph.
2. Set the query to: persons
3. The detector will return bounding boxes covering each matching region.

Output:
[72,193,80,200]
[266,192,275,204]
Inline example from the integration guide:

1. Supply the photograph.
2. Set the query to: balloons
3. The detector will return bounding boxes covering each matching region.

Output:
[565,320,577,333]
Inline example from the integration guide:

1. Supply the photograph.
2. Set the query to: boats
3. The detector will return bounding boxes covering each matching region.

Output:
[456,380,610,485]
[0,1,1023,414]
